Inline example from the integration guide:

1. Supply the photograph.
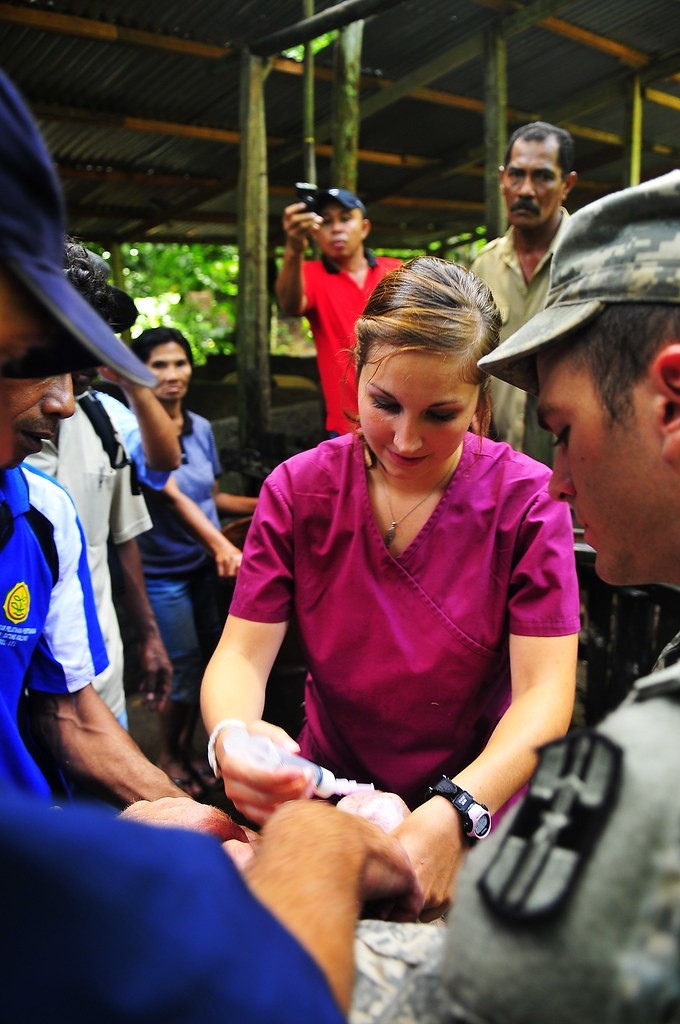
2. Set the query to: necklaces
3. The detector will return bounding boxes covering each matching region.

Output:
[384,450,457,543]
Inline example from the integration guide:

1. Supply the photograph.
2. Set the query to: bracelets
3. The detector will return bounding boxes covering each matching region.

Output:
[208,719,246,781]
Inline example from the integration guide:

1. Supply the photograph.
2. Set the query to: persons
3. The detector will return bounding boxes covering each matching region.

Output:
[1,72,680,1023]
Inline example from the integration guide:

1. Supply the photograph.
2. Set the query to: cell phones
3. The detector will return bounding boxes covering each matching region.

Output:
[296,183,317,213]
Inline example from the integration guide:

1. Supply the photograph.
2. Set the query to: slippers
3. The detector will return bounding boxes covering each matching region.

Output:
[178,738,224,792]
[158,757,207,804]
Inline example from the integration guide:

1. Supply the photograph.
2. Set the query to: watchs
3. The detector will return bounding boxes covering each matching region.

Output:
[427,774,493,850]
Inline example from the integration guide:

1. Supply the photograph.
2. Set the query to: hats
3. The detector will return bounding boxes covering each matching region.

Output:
[476,166,680,399]
[312,188,367,218]
[0,70,158,389]
